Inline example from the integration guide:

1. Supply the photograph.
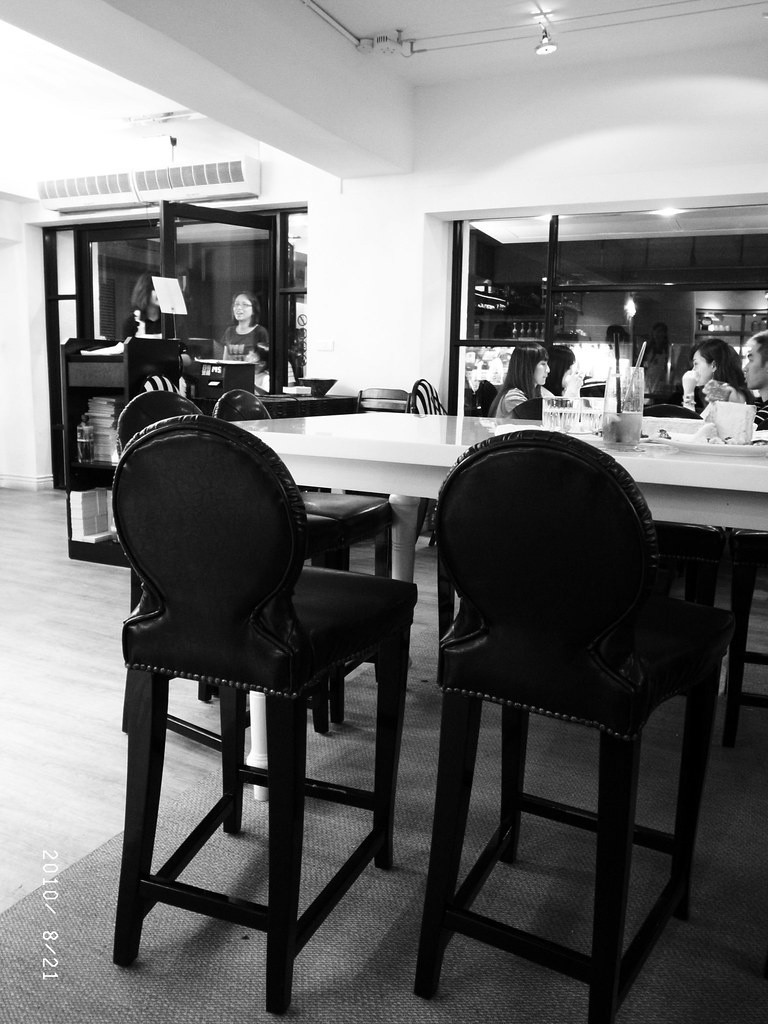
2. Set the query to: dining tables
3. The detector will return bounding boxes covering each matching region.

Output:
[227,415,768,802]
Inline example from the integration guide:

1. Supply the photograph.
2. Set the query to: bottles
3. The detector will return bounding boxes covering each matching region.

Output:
[512,322,545,339]
[76,414,95,463]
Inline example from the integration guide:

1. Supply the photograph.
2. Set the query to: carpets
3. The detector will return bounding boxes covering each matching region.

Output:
[0,628,768,1024]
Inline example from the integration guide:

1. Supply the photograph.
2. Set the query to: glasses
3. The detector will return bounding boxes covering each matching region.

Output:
[233,303,253,308]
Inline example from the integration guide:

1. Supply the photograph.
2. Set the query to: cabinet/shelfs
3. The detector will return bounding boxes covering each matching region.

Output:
[60,335,222,568]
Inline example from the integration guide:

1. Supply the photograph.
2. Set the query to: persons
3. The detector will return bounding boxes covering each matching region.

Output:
[489,342,556,418]
[642,322,680,393]
[709,331,768,431]
[542,345,583,395]
[123,273,162,340]
[683,339,755,415]
[220,290,270,395]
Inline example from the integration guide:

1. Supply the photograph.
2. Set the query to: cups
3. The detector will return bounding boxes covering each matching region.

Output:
[542,398,603,433]
[603,366,645,450]
[705,380,730,403]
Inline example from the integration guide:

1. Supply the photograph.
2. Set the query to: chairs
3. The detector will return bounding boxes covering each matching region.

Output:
[112,378,768,1024]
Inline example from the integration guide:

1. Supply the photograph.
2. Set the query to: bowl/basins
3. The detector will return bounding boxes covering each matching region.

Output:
[298,378,337,397]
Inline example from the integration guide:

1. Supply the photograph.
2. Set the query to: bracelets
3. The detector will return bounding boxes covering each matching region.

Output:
[684,394,696,405]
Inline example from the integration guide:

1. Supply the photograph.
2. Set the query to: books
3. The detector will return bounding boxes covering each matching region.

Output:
[84,398,125,461]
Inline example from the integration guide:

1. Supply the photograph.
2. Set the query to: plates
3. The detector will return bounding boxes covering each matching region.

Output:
[653,433,768,456]
